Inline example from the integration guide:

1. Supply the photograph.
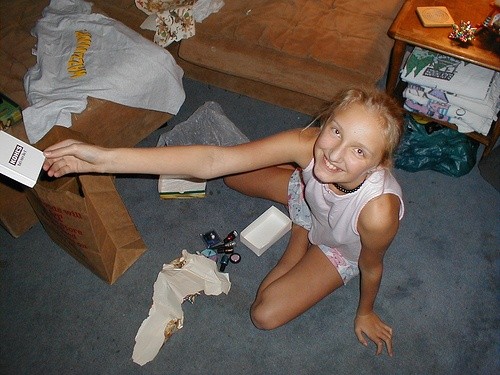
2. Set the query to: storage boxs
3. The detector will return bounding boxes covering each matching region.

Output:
[239,205,292,256]
[159,177,206,200]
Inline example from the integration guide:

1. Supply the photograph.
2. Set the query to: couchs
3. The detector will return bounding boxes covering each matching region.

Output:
[0,0,406,240]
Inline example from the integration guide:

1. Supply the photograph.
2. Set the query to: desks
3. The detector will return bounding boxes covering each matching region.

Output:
[386,0,500,162]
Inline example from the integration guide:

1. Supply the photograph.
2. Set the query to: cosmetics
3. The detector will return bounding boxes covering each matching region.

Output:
[195,228,242,272]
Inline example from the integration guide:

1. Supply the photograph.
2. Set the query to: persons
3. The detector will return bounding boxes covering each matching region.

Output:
[37,85,409,359]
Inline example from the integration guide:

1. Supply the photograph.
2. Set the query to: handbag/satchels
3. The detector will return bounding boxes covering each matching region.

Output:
[392,113,479,178]
[6,120,148,286]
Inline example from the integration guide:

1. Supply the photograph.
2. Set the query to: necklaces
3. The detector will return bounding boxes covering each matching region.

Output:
[324,181,365,193]
